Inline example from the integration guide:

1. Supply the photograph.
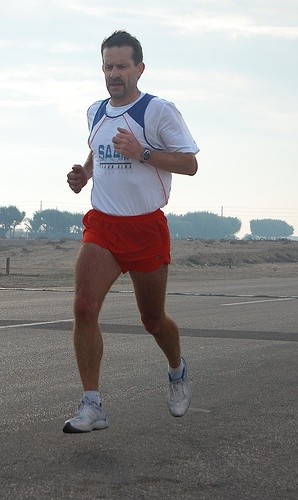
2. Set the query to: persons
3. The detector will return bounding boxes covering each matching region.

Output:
[61,30,201,433]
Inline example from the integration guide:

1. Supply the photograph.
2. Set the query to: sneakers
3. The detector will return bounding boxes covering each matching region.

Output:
[63,396,109,433]
[167,356,191,417]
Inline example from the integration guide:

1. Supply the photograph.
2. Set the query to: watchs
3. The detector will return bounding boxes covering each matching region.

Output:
[139,148,154,163]
